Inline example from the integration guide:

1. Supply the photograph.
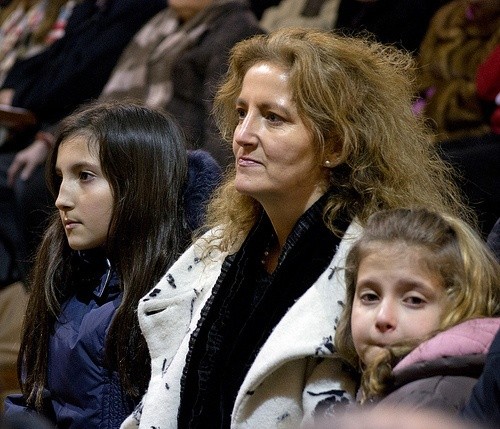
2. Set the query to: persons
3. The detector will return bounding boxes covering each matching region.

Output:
[340,203,499,429]
[0,99,231,429]
[115,31,466,429]
[0,0,499,290]
